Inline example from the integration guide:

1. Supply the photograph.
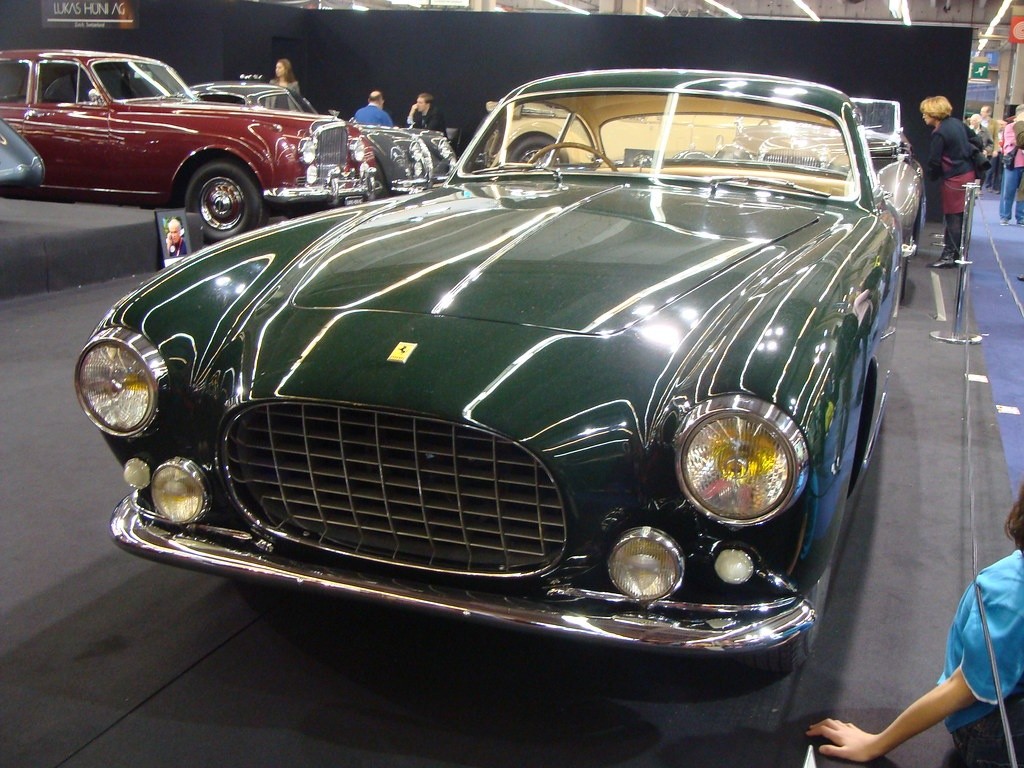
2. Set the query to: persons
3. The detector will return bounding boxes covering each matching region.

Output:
[354,91,393,127]
[920,96,983,268]
[268,59,300,94]
[166,219,188,256]
[405,93,449,140]
[964,103,1024,227]
[804,484,1023,768]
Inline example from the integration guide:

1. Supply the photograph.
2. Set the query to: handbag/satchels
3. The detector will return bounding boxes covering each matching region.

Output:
[1002,155,1015,170]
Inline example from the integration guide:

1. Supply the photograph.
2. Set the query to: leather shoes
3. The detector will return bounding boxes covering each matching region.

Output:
[925,257,960,268]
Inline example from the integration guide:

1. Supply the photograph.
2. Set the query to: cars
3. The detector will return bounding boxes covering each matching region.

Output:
[2,47,376,245]
[483,94,928,247]
[79,65,908,683]
[174,81,456,196]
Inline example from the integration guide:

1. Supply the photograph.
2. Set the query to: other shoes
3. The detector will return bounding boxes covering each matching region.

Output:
[987,187,993,193]
[995,190,1000,194]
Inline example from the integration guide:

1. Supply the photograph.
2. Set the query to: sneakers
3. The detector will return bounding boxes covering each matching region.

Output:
[1017,221,1024,227]
[1000,218,1009,226]
